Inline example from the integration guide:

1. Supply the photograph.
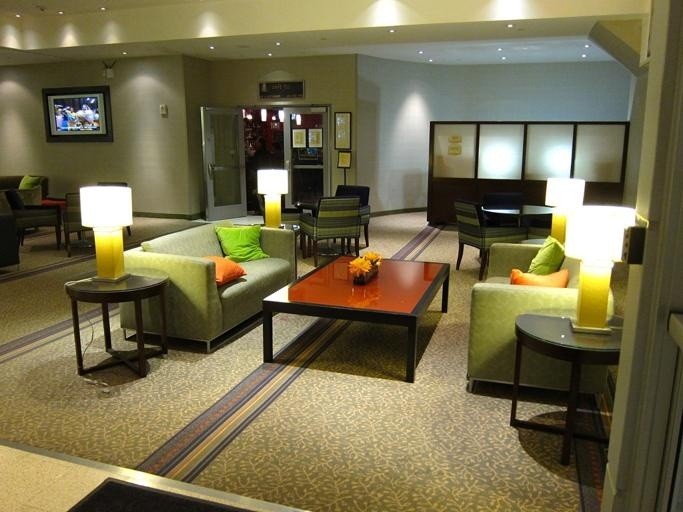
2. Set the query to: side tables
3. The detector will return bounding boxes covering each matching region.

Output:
[64,267,170,376]
[510,309,625,467]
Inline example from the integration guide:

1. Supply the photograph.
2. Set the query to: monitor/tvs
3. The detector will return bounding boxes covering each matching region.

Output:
[47,94,107,135]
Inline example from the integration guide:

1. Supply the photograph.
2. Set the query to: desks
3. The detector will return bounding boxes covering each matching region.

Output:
[481,204,553,225]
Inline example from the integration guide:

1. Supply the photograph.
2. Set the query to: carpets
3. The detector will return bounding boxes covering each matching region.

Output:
[66,476,252,511]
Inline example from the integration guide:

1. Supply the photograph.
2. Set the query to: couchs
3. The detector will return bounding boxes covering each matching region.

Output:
[463,236,614,395]
[120,219,295,354]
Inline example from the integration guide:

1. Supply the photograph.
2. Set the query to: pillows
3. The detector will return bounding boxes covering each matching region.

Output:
[213,225,268,262]
[509,266,569,289]
[525,236,566,275]
[201,255,249,286]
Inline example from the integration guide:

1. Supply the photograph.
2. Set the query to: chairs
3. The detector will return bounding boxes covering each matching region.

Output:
[0,169,98,257]
[453,199,526,281]
[481,191,522,227]
[294,183,369,268]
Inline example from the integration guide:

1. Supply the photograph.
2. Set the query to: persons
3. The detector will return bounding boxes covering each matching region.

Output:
[54,103,100,131]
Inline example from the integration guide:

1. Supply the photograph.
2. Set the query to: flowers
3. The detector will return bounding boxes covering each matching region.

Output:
[346,251,382,277]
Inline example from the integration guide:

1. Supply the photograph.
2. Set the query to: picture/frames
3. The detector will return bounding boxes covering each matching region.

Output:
[257,80,305,99]
[40,85,114,144]
[289,110,353,170]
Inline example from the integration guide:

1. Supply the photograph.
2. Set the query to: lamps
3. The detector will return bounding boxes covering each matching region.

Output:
[543,177,587,245]
[80,185,132,281]
[563,202,641,334]
[257,170,289,231]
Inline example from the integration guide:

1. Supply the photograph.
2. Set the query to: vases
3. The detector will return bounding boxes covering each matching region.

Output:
[353,266,379,283]
